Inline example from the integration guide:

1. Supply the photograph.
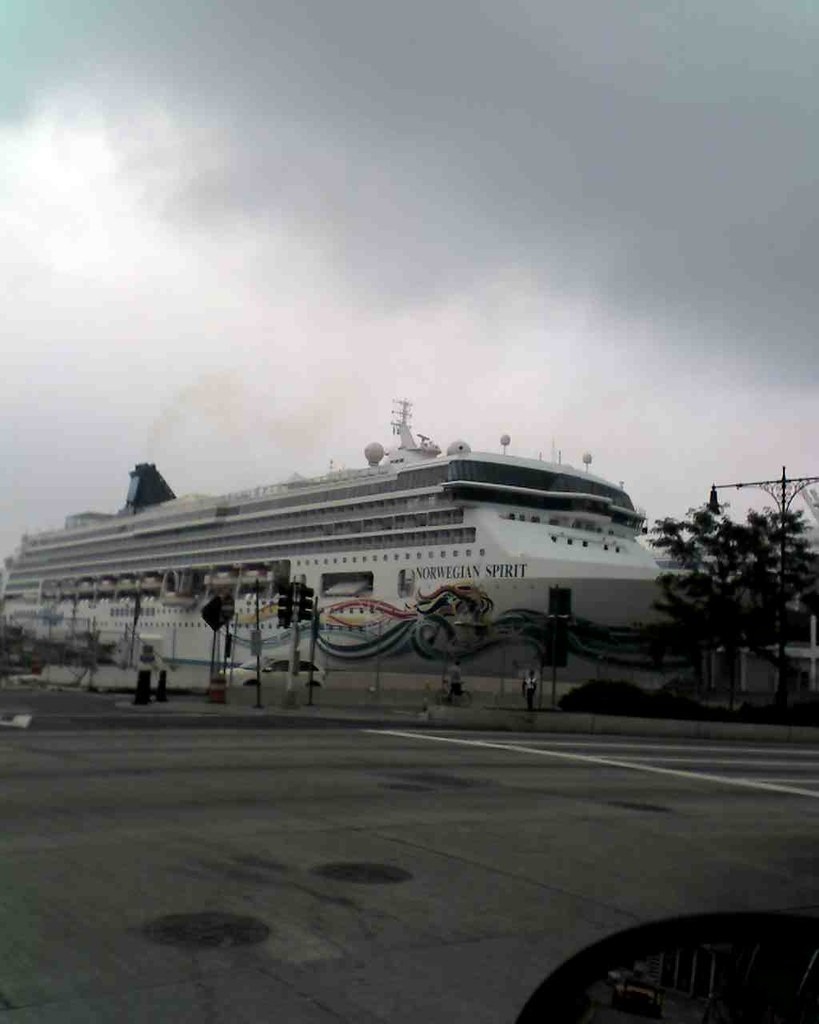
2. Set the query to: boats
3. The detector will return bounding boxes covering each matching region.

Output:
[76,581,92,598]
[61,584,74,598]
[203,571,237,593]
[97,579,115,596]
[241,569,272,591]
[118,578,137,595]
[141,575,161,594]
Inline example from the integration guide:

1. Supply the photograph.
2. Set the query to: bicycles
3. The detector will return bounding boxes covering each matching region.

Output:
[435,680,474,708]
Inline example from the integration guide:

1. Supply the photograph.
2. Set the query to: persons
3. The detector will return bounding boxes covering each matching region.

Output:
[450,661,461,705]
[526,671,536,710]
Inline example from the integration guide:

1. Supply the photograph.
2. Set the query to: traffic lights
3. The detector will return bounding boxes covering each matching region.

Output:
[296,583,314,620]
[277,583,294,619]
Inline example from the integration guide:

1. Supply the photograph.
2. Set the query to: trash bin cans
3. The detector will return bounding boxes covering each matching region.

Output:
[133,671,151,705]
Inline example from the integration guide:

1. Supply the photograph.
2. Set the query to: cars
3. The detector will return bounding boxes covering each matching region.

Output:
[225,654,327,690]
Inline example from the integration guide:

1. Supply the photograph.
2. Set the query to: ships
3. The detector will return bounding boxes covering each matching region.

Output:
[0,400,665,677]
